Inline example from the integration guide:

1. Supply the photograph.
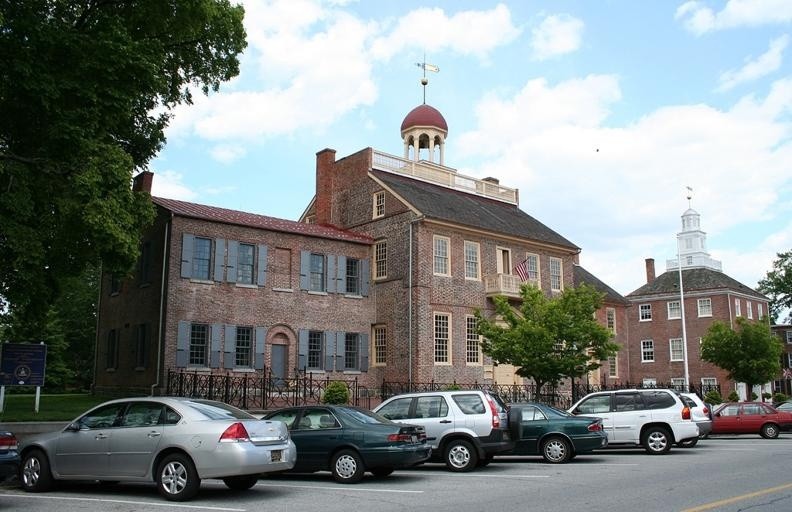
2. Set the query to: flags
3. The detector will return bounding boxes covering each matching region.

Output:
[515,259,529,282]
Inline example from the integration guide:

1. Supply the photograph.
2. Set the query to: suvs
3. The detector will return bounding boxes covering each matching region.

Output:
[371,391,523,476]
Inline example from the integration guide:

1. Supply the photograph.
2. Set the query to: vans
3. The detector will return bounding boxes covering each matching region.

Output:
[566,388,713,454]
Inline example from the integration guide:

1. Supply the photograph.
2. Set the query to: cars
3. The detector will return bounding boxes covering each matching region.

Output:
[503,402,608,463]
[0,431,22,482]
[699,401,792,442]
[256,406,431,485]
[18,395,296,499]
